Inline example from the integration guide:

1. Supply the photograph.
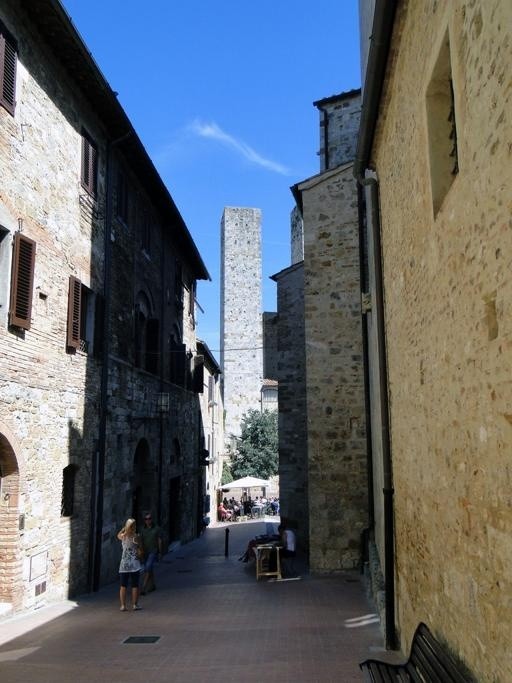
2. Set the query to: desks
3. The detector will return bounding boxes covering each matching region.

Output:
[252,540,284,582]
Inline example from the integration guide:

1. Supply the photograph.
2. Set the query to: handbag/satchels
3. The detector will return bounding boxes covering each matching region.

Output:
[137,545,145,560]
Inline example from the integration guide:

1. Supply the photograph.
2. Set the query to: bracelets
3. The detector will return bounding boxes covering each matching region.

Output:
[159,551,162,553]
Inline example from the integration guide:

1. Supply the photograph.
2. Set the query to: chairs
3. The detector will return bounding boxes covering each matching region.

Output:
[218,501,279,522]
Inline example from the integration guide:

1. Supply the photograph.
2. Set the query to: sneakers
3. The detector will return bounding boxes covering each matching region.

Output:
[140,584,155,594]
[132,605,142,611]
[120,606,129,611]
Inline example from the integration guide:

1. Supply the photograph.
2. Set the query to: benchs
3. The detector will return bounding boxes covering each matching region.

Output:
[358,622,467,683]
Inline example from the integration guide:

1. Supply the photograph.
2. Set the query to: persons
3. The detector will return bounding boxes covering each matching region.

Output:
[116,517,144,611]
[218,491,281,521]
[276,523,298,579]
[135,512,166,596]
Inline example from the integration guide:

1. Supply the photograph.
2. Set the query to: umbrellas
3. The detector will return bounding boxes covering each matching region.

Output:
[218,474,273,501]
